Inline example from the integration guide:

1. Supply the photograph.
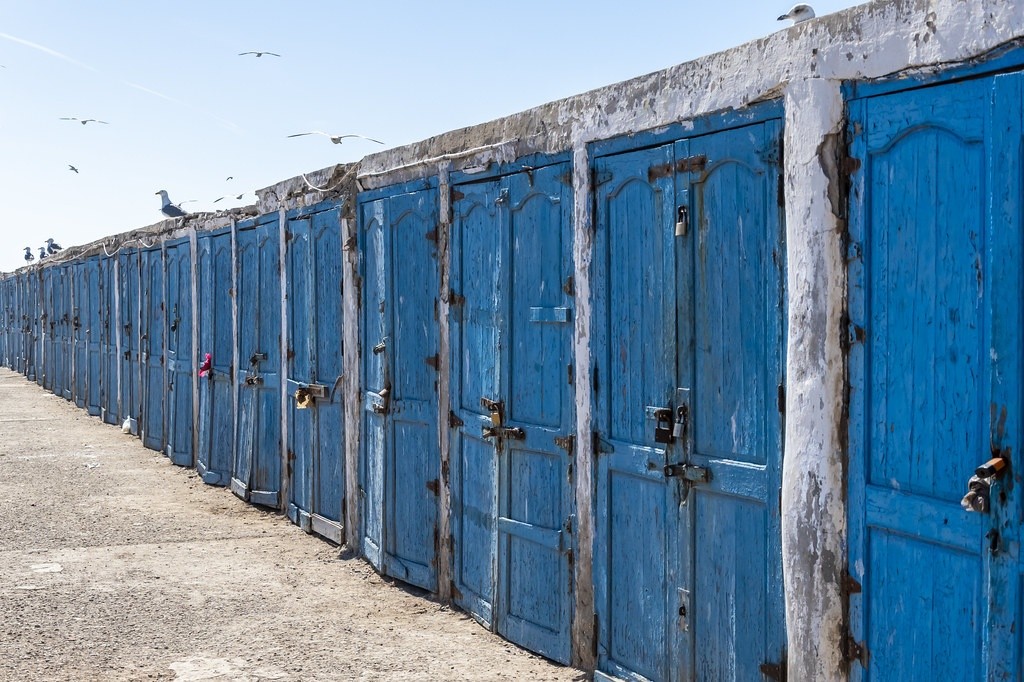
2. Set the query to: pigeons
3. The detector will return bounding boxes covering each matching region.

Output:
[23,237,64,266]
[155,190,191,218]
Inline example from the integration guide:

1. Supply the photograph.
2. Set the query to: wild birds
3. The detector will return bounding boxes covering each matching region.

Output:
[287,131,384,145]
[59,117,109,125]
[121,415,131,434]
[238,51,281,57]
[158,176,245,211]
[69,165,78,173]
[777,3,815,26]
